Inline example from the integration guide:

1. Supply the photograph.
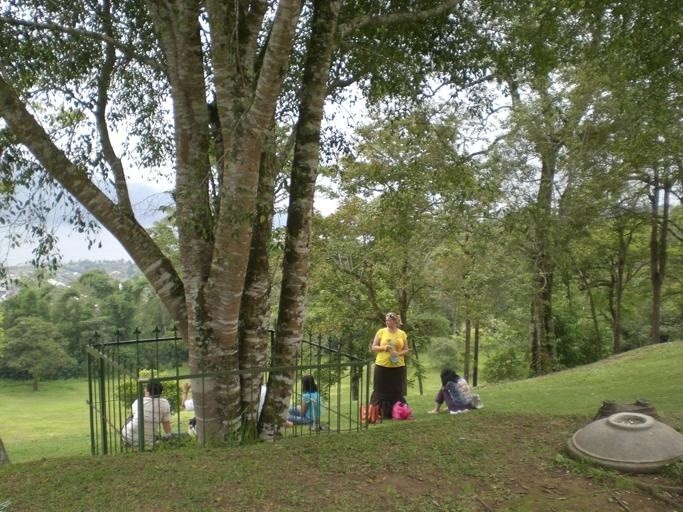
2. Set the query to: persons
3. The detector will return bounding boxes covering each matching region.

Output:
[370,312,408,419]
[182,383,197,438]
[428,368,473,414]
[287,375,322,424]
[121,382,171,448]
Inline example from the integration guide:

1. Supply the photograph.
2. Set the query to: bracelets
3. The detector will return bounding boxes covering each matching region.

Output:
[183,392,188,394]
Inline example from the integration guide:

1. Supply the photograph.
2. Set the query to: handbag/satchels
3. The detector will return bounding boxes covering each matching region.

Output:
[472,393,483,409]
[392,401,411,420]
[360,398,381,423]
